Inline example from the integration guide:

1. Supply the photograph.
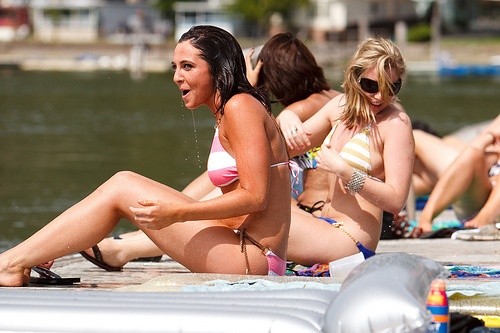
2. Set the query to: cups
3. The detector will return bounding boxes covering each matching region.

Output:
[329,251,366,279]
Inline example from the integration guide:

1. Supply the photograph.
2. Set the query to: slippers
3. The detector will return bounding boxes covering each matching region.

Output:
[114,235,163,263]
[27,266,82,284]
[79,242,123,272]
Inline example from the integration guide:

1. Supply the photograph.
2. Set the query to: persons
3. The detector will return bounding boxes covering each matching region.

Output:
[77,39,414,274]
[181,34,353,215]
[0,26,294,287]
[405,117,500,244]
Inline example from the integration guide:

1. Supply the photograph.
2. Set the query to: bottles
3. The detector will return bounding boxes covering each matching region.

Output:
[426,278,451,333]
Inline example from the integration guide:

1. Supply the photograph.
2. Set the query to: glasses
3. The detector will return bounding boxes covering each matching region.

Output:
[359,77,403,96]
